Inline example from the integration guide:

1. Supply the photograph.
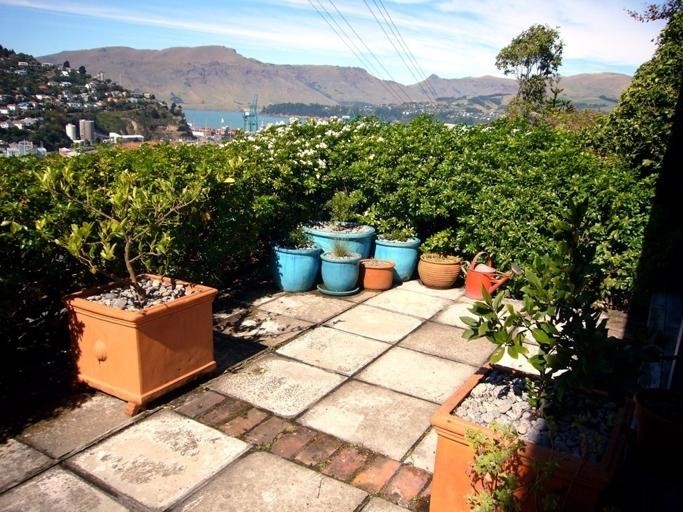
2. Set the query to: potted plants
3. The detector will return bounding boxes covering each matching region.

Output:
[416,196,637,512]
[0,151,248,420]
[270,188,466,297]
